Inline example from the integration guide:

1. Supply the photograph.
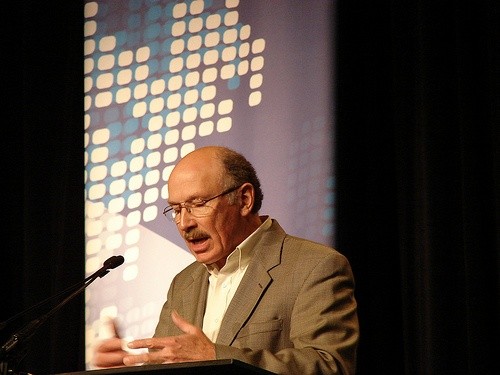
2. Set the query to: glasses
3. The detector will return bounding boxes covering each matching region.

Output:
[163,184,241,223]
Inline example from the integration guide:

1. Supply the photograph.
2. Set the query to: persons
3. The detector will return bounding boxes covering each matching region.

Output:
[94,146,360,374]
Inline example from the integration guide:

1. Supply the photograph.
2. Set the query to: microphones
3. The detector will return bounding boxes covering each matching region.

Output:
[0,256,124,356]
